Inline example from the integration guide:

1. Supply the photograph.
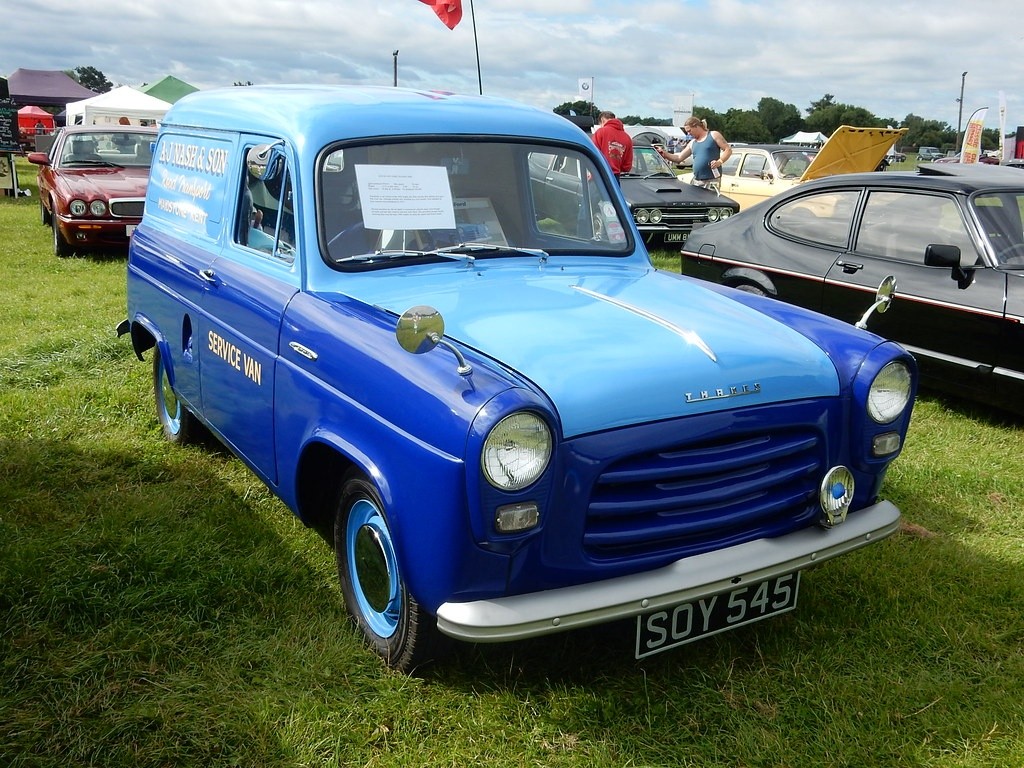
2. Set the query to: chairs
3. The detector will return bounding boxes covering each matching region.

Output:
[979,204,1018,258]
[65,141,103,161]
[423,170,526,253]
[133,140,155,163]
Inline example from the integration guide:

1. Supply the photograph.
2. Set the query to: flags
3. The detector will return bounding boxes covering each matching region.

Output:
[419,0,463,31]
[579,76,592,100]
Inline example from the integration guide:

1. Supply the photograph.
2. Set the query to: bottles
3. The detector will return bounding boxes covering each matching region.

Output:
[711,161,720,179]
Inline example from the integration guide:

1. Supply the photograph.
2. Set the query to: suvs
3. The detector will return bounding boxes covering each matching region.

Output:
[917,146,946,162]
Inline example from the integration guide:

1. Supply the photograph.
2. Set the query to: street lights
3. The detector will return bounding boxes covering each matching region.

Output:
[392,49,400,89]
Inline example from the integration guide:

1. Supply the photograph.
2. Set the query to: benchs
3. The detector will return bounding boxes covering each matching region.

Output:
[884,194,941,258]
[63,140,141,164]
[787,199,854,242]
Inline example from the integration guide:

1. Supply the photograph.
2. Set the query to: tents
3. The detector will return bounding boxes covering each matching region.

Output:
[778,130,829,150]
[6,68,201,159]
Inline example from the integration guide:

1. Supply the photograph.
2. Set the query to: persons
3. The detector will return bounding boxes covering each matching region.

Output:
[34,120,46,135]
[575,110,634,241]
[654,116,731,194]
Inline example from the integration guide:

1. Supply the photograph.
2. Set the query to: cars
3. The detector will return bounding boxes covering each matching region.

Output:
[116,82,924,674]
[28,123,164,256]
[1004,158,1024,170]
[675,143,879,220]
[682,161,1024,420]
[524,135,741,249]
[886,150,908,163]
[934,151,1000,165]
